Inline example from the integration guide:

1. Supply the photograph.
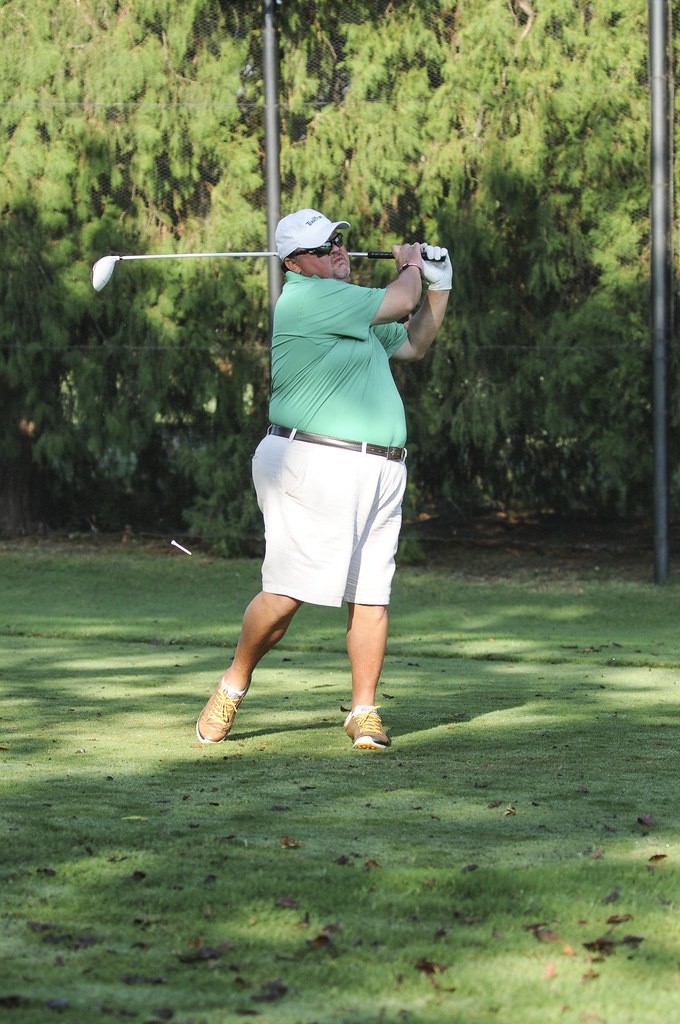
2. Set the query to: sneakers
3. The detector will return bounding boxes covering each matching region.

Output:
[196,668,253,746]
[341,707,393,750]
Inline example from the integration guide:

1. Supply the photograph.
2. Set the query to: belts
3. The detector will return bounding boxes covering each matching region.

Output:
[267,423,405,463]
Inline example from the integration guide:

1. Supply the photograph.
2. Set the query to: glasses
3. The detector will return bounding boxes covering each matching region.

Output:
[289,233,346,257]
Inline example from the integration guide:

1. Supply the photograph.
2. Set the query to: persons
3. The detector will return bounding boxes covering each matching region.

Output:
[196,209,452,750]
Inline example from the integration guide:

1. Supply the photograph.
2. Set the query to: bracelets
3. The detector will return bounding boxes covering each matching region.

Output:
[399,262,422,272]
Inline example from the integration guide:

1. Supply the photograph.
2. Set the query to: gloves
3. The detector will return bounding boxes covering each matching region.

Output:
[414,241,453,292]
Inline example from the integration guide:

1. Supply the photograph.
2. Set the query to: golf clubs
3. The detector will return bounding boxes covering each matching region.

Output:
[89,252,447,292]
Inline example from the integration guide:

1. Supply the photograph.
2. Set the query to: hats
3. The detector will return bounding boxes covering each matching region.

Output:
[274,207,352,260]
